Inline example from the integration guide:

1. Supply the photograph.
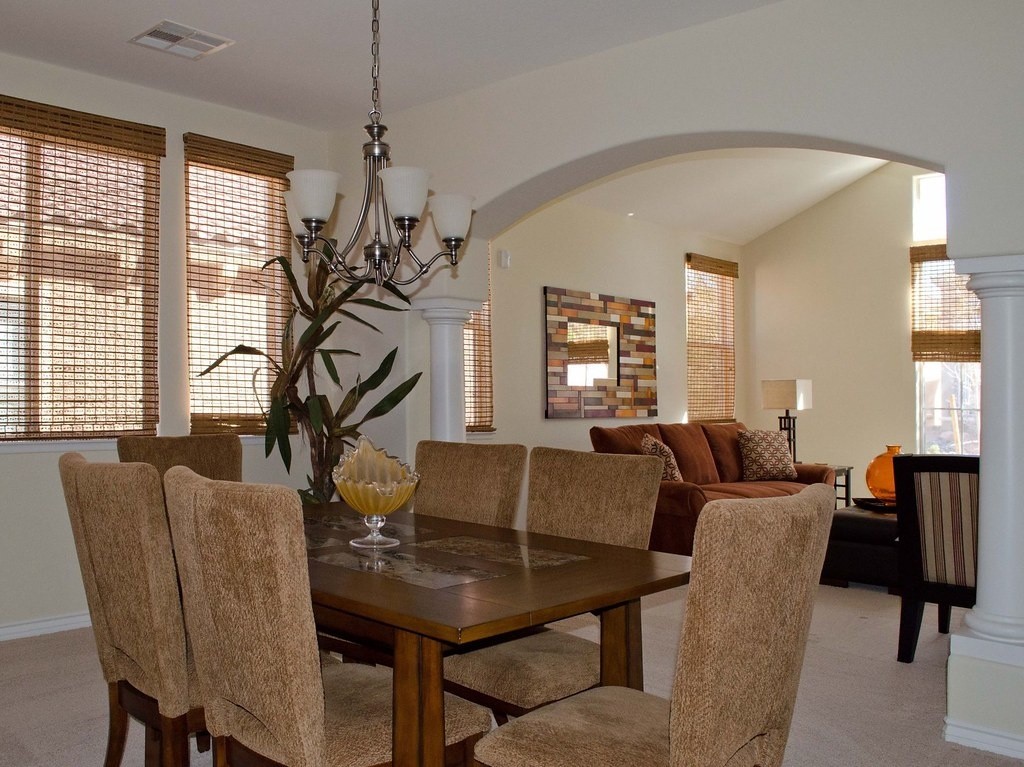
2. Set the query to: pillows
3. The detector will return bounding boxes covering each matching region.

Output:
[736,428,798,481]
[641,432,684,482]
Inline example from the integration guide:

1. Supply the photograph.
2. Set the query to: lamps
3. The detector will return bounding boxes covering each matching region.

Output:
[762,379,812,465]
[282,0,476,286]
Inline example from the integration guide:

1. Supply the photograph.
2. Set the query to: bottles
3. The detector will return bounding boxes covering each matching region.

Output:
[865,444,905,499]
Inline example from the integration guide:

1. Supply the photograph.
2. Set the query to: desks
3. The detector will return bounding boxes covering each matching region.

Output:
[818,505,898,595]
[303,501,692,767]
[813,463,854,511]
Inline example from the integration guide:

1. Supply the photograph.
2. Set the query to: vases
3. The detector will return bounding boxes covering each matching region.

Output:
[866,444,903,506]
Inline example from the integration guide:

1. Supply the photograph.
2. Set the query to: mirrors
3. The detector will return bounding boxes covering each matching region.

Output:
[567,316,620,387]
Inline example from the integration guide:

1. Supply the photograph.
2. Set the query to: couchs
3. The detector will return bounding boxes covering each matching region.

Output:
[591,419,835,557]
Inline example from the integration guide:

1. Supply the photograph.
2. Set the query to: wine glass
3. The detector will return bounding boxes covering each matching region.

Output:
[331,434,420,549]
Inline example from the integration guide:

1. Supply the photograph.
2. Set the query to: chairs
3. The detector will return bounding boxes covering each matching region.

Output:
[413,439,528,529]
[117,433,243,753]
[475,482,836,767]
[892,454,979,664]
[58,452,207,767]
[441,447,665,727]
[164,465,493,767]
